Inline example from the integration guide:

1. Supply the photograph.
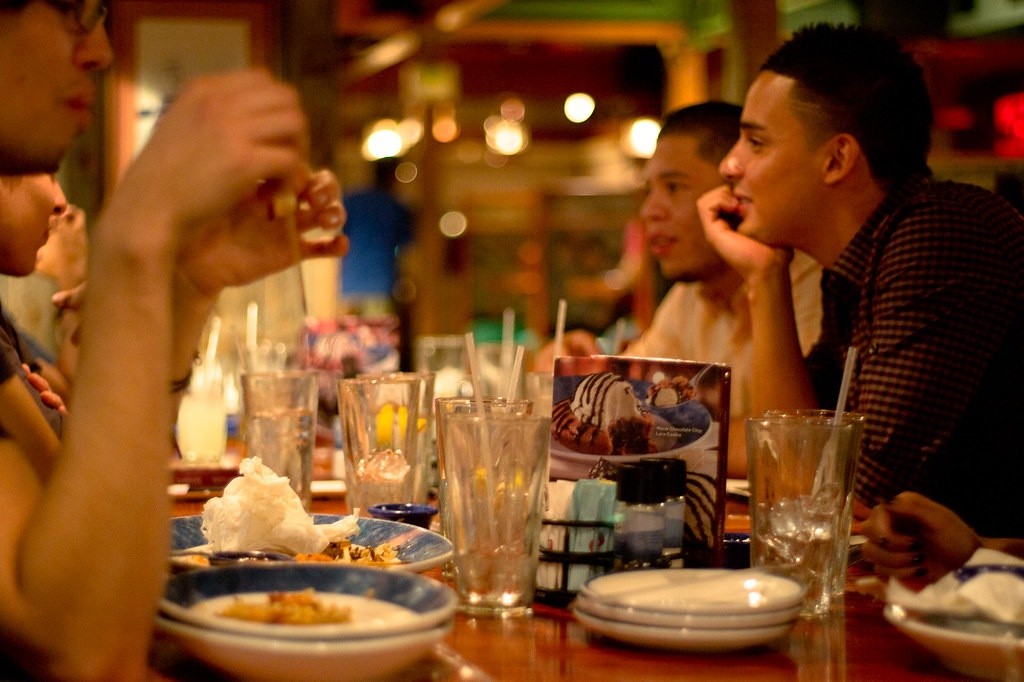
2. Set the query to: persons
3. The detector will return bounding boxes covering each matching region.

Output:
[341,156,413,374]
[697,23,1024,539]
[862,491,1024,583]
[558,100,820,478]
[0,0,349,682]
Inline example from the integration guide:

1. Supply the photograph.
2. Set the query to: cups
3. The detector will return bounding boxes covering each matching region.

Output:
[240,370,316,512]
[336,378,419,518]
[176,366,227,462]
[440,411,553,621]
[744,416,855,626]
[759,409,864,612]
[355,371,436,506]
[367,502,438,532]
[433,395,534,582]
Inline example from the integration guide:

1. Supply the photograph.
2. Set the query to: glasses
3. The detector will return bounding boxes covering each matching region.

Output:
[47,0,107,33]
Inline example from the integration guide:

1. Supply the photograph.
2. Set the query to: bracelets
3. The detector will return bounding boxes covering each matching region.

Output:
[169,372,195,392]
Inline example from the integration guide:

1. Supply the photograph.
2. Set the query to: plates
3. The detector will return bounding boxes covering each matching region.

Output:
[880,584,1024,682]
[156,564,461,641]
[150,615,456,682]
[165,513,457,576]
[570,567,806,654]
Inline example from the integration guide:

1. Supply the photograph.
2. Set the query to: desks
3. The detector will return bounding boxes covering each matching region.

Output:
[173,495,956,682]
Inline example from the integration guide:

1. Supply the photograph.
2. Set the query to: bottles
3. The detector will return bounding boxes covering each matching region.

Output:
[660,460,688,547]
[614,456,664,569]
